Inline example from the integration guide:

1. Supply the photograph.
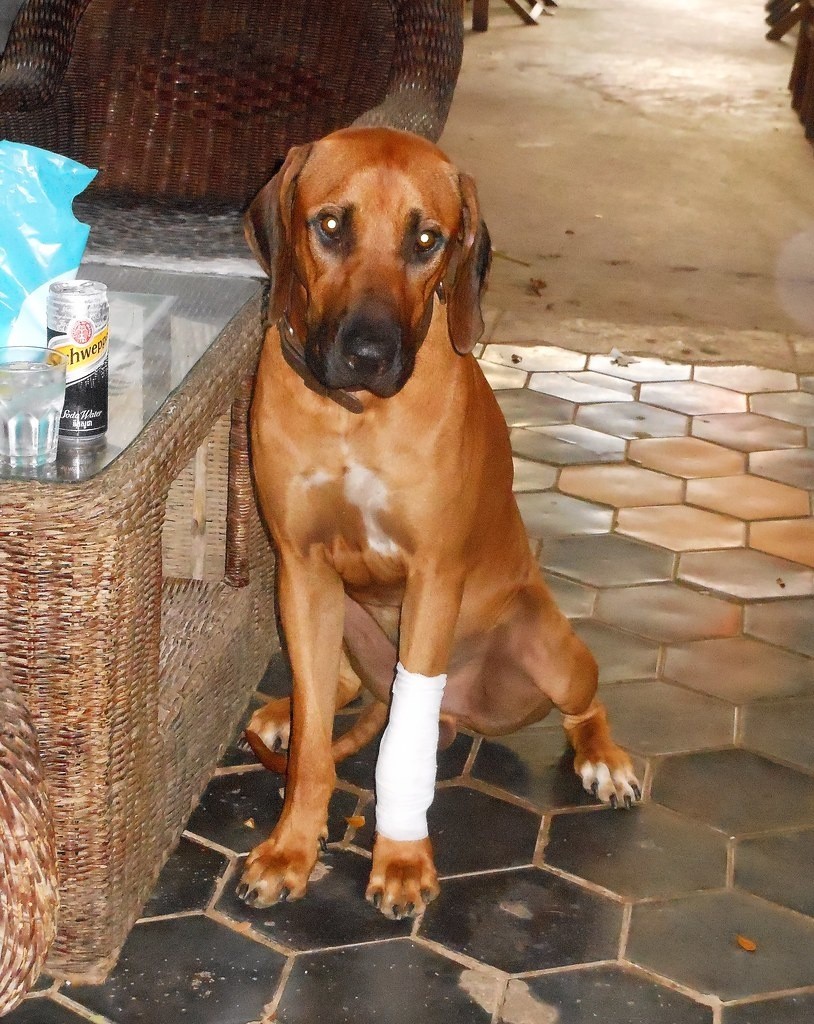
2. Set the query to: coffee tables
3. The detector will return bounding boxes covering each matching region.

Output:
[0,263,281,986]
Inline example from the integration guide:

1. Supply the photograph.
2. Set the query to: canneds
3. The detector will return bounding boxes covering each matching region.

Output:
[46,280,110,442]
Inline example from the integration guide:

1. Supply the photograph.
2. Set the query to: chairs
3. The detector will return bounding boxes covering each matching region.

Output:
[0,1,465,277]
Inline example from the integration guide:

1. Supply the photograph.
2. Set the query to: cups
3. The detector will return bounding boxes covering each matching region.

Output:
[0,346,68,457]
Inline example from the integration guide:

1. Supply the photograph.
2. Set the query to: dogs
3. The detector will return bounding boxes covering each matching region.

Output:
[233,126,642,925]
